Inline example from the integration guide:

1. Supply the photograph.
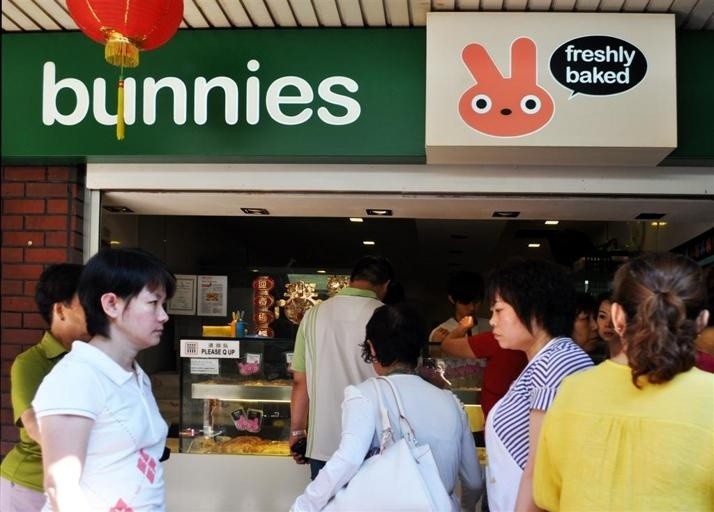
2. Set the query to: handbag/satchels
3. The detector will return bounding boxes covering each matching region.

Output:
[318,374,454,512]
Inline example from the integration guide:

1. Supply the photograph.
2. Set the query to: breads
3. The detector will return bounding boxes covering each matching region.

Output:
[221,435,291,454]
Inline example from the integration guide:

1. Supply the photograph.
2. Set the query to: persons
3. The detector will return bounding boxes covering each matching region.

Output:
[484,252,714,511]
[32,245,179,512]
[288,255,528,511]
[0,262,91,510]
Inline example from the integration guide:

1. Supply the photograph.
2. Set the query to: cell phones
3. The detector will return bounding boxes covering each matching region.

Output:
[292,438,311,464]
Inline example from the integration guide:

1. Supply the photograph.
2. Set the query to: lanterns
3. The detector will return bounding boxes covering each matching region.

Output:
[63,2,182,141]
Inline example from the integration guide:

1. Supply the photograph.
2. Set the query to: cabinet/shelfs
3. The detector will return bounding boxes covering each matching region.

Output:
[180,379,488,512]
